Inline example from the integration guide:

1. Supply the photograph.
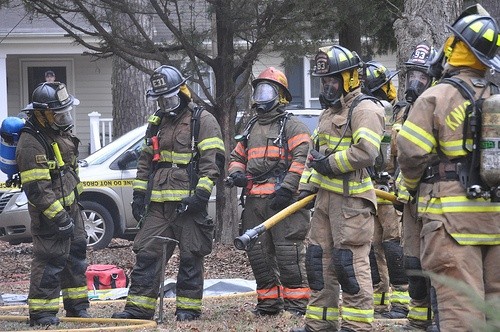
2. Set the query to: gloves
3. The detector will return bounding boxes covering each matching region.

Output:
[176,190,209,215]
[268,188,293,211]
[57,219,75,241]
[297,189,313,211]
[230,171,247,188]
[132,190,146,223]
[306,151,334,175]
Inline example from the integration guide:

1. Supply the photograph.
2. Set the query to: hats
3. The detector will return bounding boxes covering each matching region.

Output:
[46,71,55,75]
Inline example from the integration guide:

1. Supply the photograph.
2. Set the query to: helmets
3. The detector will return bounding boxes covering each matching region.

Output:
[313,44,360,76]
[357,60,402,93]
[20,82,74,111]
[406,40,434,66]
[146,65,192,96]
[250,67,293,102]
[446,13,500,72]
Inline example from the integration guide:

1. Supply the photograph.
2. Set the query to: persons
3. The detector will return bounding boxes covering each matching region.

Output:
[15,82,92,326]
[111,65,225,321]
[395,4,500,332]
[291,44,386,332]
[362,40,447,332]
[227,68,313,315]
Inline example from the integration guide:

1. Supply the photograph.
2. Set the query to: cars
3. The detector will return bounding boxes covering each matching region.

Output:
[0,109,324,251]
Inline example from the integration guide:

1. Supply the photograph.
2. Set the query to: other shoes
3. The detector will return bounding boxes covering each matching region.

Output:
[427,322,439,332]
[64,309,91,318]
[29,314,60,327]
[175,312,194,323]
[382,311,405,319]
[291,327,306,332]
[111,310,134,319]
